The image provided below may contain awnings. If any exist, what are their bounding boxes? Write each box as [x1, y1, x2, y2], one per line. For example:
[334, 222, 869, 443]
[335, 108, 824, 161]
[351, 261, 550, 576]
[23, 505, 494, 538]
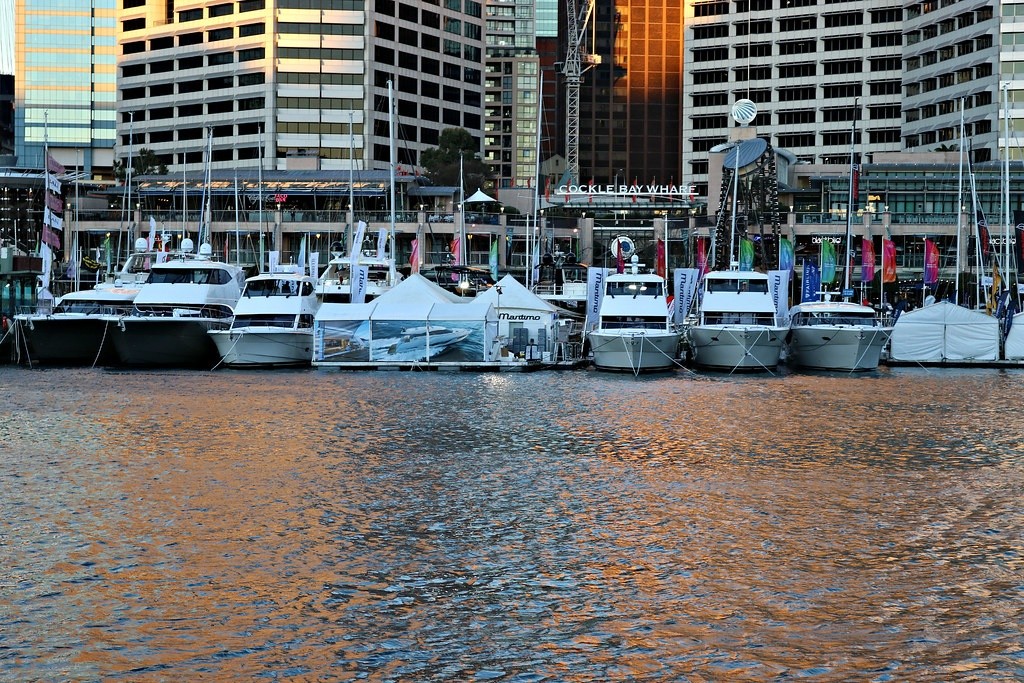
[136, 181, 392, 194]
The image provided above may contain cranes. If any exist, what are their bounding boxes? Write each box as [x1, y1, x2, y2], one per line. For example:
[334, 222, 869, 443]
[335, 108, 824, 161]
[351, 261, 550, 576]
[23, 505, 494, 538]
[560, 0, 604, 191]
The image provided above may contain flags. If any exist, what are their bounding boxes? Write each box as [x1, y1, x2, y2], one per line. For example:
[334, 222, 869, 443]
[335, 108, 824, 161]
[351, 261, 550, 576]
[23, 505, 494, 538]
[42, 153, 63, 291]
[67, 244, 101, 277]
[495, 176, 695, 203]
[224, 238, 228, 256]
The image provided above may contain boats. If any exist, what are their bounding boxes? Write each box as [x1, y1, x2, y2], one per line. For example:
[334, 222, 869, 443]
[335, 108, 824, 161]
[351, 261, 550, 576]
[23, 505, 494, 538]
[585, 270, 678, 372]
[206, 274, 322, 367]
[98, 256, 243, 372]
[324, 334, 361, 358]
[389, 325, 472, 355]
[684, 143, 791, 369]
[315, 256, 405, 300]
[785, 300, 895, 373]
[12, 242, 209, 364]
[532, 253, 588, 312]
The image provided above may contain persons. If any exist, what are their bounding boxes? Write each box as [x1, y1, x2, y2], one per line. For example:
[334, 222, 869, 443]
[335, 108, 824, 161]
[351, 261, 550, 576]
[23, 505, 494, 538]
[281, 281, 313, 297]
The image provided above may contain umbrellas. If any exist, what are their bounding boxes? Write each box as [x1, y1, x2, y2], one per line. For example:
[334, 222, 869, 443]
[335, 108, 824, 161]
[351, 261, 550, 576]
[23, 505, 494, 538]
[460, 187, 500, 203]
